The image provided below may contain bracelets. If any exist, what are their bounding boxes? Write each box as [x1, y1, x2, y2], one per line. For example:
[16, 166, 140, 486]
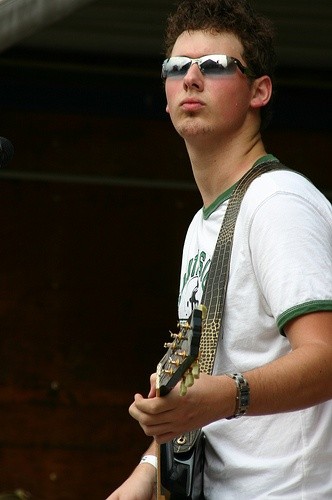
[139, 455, 158, 470]
[224, 372, 251, 421]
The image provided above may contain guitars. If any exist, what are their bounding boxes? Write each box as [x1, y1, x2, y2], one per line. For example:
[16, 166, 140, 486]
[155, 309, 202, 500]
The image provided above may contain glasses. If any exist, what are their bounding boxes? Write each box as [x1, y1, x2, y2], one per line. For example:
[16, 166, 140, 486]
[161, 54, 256, 79]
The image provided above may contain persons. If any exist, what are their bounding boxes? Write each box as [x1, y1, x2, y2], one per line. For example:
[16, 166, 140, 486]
[104, 0, 332, 500]
